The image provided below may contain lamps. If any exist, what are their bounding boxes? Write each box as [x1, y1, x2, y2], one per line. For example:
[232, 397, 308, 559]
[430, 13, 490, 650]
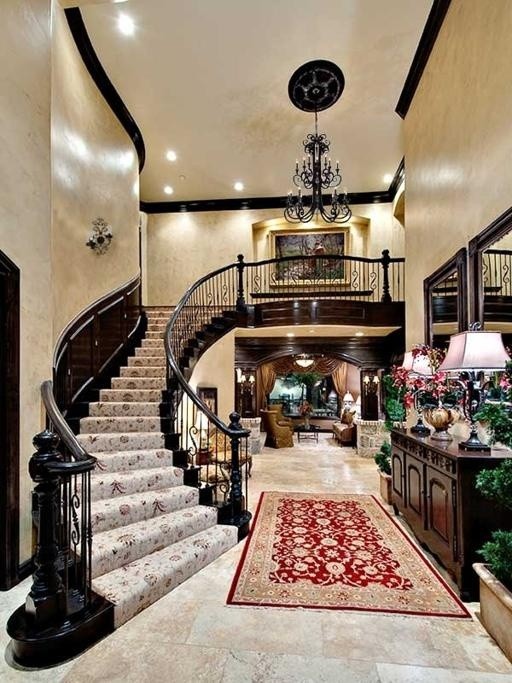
[236, 368, 255, 396]
[194, 410, 210, 451]
[436, 321, 512, 452]
[294, 354, 316, 368]
[343, 389, 355, 407]
[85, 215, 113, 254]
[363, 375, 370, 396]
[401, 350, 436, 436]
[284, 58, 352, 224]
[372, 376, 380, 393]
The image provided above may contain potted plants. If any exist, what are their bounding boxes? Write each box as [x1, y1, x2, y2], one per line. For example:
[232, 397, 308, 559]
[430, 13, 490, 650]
[373, 440, 392, 505]
[471, 457, 512, 665]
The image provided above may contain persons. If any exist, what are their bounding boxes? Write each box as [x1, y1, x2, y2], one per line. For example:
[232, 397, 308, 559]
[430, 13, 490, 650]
[275, 237, 344, 278]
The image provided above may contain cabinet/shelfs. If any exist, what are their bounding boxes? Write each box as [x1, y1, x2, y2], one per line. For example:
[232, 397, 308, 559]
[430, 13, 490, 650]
[389, 429, 512, 603]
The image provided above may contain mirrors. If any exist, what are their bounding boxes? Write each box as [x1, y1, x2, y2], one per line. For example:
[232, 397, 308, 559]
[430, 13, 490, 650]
[469, 204, 512, 358]
[423, 246, 468, 357]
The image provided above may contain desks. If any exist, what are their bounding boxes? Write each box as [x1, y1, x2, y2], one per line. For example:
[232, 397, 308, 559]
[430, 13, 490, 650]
[196, 449, 214, 465]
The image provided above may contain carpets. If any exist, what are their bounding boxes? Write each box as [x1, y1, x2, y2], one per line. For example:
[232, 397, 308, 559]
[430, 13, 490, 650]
[227, 491, 472, 620]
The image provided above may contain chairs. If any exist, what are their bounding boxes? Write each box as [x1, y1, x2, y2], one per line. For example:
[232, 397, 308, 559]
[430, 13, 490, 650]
[208, 426, 252, 479]
[260, 409, 294, 449]
[267, 404, 294, 435]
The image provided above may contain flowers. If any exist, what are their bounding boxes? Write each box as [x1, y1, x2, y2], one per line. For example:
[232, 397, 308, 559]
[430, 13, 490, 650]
[390, 344, 512, 409]
[297, 401, 313, 417]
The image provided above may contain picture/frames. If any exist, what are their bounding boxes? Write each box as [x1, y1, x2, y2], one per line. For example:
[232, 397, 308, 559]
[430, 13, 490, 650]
[269, 225, 352, 288]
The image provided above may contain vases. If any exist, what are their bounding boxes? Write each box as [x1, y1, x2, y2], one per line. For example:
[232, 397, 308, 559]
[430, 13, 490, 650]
[423, 407, 454, 442]
[304, 415, 311, 431]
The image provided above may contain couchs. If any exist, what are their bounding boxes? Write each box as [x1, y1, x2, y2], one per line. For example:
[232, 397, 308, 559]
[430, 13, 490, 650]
[332, 412, 355, 449]
